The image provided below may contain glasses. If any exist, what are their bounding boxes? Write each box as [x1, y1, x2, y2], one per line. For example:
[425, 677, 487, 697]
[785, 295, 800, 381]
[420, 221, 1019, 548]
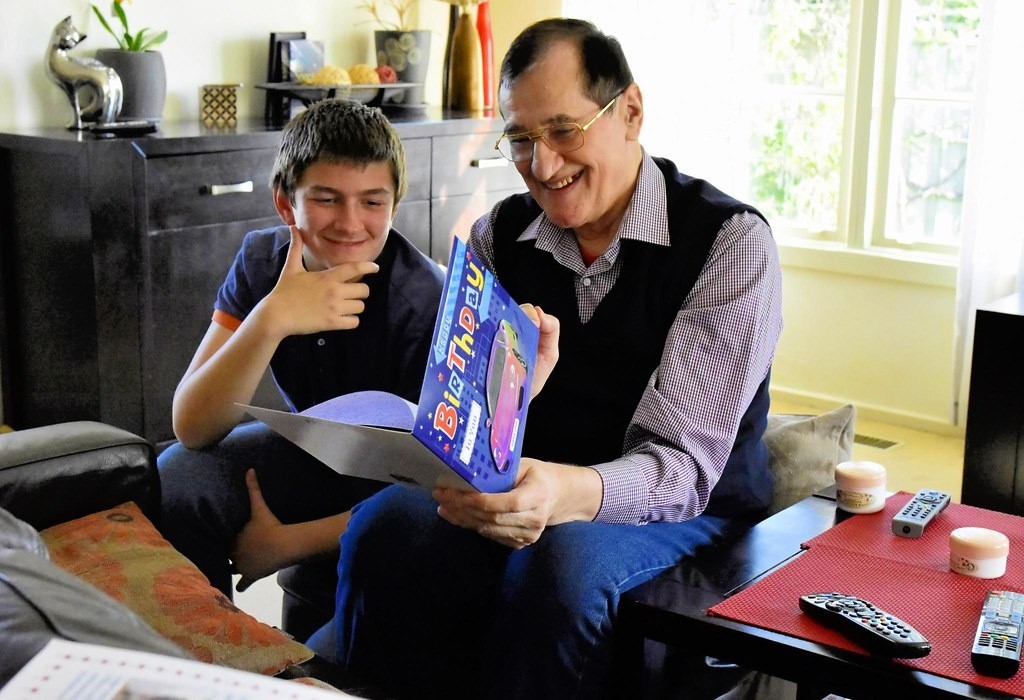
[495, 90, 624, 162]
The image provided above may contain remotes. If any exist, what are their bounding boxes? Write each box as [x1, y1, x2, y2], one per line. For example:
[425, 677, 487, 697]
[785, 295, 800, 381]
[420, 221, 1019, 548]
[971, 591, 1024, 679]
[799, 593, 932, 660]
[891, 489, 952, 537]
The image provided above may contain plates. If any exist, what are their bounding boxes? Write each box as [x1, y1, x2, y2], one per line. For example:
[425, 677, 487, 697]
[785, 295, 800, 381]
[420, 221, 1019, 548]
[255, 81, 421, 106]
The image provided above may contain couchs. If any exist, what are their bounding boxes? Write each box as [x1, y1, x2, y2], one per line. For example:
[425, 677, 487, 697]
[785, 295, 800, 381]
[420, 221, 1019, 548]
[0, 421, 303, 699]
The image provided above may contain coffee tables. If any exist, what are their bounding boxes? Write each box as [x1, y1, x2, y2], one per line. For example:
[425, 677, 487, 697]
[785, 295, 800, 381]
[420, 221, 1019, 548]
[619, 484, 1024, 700]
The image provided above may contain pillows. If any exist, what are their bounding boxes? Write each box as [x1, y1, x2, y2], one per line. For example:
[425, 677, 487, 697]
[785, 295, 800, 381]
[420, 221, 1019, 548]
[763, 403, 857, 516]
[42, 500, 317, 676]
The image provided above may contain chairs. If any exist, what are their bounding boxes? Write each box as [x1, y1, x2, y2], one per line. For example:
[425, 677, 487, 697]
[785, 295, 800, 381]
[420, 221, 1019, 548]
[958, 309, 1024, 518]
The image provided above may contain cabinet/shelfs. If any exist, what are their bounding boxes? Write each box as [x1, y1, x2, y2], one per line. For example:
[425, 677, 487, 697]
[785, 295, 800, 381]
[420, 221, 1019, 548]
[0, 115, 531, 444]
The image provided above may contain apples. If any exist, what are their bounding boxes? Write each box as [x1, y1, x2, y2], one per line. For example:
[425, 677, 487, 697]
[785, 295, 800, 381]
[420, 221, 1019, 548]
[375, 64, 397, 83]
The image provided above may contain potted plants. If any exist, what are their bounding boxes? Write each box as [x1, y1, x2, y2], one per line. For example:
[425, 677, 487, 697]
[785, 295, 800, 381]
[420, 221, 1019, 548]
[90, 0, 168, 123]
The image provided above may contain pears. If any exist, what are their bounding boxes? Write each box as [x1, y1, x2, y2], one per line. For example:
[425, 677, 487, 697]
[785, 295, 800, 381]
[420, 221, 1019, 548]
[311, 64, 351, 86]
[348, 64, 380, 84]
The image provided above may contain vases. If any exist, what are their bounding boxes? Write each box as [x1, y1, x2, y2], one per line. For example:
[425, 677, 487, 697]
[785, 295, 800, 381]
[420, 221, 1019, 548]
[374, 31, 435, 113]
[448, 12, 485, 109]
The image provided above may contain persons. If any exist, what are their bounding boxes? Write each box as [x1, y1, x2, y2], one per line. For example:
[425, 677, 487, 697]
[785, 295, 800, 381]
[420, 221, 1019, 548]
[337, 19, 783, 700]
[158, 99, 445, 644]
